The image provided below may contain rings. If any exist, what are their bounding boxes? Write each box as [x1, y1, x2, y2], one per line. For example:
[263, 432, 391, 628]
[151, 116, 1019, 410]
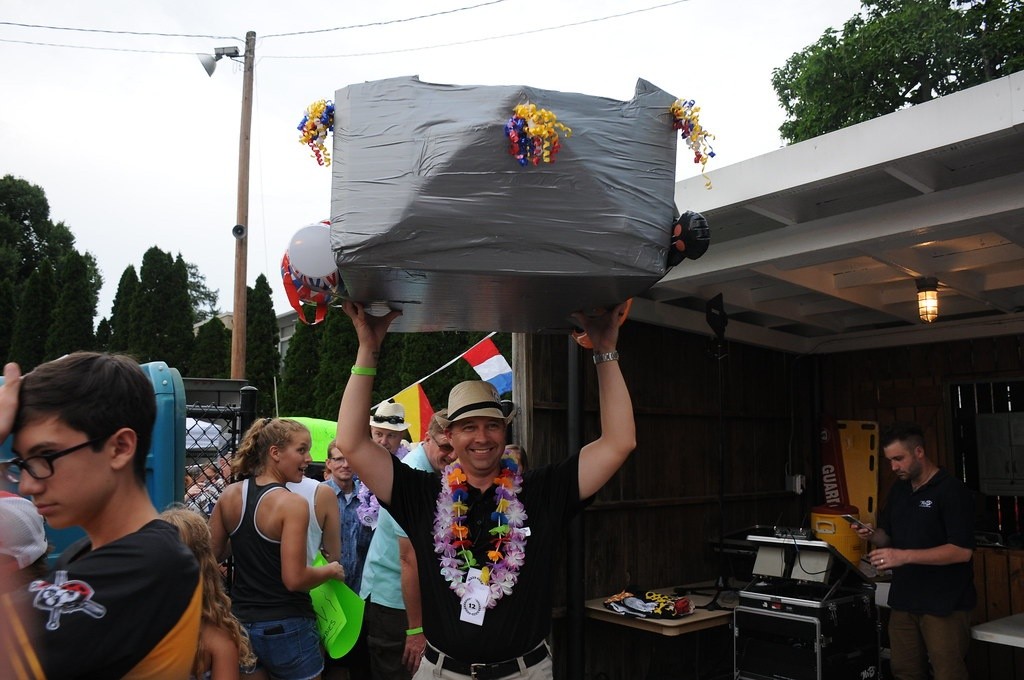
[881, 559, 883, 563]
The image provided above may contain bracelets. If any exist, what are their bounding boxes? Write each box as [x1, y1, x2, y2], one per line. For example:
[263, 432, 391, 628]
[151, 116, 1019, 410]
[593, 351, 619, 363]
[406, 627, 423, 636]
[350, 365, 377, 376]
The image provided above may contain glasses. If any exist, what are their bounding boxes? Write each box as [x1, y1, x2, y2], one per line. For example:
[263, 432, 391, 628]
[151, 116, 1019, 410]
[0, 429, 143, 484]
[428, 432, 454, 453]
[330, 456, 345, 465]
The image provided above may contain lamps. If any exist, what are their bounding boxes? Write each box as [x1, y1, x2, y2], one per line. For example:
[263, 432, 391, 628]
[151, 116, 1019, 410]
[914, 276, 940, 323]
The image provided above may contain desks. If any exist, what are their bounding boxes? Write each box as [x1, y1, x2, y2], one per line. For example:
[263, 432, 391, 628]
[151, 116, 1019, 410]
[970, 613, 1024, 649]
[586, 578, 749, 680]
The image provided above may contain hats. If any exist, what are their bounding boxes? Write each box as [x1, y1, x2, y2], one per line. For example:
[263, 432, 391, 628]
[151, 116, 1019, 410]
[0, 491, 48, 578]
[370, 400, 411, 431]
[434, 380, 516, 430]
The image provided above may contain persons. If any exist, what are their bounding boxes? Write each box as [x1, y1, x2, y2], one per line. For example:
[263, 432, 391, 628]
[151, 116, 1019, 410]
[187, 437, 238, 519]
[335, 301, 636, 680]
[0, 352, 203, 680]
[285, 475, 341, 567]
[208, 416, 345, 680]
[851, 424, 978, 680]
[359, 408, 459, 680]
[320, 439, 361, 584]
[357, 401, 411, 592]
[161, 510, 258, 680]
[506, 444, 528, 474]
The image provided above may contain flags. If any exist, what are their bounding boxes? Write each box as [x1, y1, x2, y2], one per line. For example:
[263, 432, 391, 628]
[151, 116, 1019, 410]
[463, 336, 512, 395]
[394, 383, 435, 443]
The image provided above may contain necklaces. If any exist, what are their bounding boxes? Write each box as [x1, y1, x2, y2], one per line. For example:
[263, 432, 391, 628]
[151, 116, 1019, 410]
[434, 449, 528, 609]
[356, 445, 408, 526]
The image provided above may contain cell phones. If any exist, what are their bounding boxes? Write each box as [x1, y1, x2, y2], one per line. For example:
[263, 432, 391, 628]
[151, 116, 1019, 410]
[263, 624, 285, 636]
[841, 514, 875, 533]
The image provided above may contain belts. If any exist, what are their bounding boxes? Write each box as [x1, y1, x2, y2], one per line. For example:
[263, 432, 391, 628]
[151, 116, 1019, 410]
[423, 640, 550, 680]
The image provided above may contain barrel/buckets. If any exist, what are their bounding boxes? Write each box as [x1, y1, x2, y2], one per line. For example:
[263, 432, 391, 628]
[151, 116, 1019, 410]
[811, 512, 867, 568]
